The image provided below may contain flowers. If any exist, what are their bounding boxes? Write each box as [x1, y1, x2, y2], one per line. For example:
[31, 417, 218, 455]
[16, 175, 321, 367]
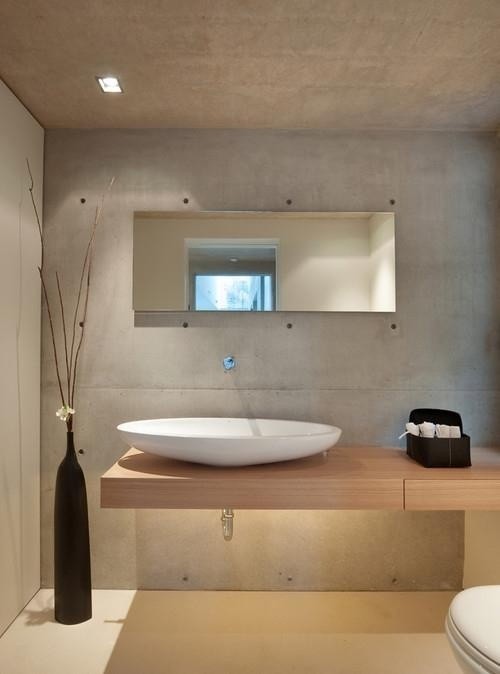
[29, 157, 117, 425]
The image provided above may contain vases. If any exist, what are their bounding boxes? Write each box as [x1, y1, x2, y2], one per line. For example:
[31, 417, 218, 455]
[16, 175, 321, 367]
[54, 432, 91, 625]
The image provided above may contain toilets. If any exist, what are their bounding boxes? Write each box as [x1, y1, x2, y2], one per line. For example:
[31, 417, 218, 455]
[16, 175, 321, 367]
[444, 583, 500, 674]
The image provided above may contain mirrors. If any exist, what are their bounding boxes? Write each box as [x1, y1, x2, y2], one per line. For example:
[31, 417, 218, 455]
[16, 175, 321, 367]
[132, 211, 396, 313]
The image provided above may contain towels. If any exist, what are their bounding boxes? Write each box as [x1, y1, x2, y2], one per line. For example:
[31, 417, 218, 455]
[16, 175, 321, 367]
[405, 421, 461, 439]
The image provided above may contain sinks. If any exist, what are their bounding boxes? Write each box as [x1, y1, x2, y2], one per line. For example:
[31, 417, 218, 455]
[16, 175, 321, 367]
[116, 417, 343, 467]
